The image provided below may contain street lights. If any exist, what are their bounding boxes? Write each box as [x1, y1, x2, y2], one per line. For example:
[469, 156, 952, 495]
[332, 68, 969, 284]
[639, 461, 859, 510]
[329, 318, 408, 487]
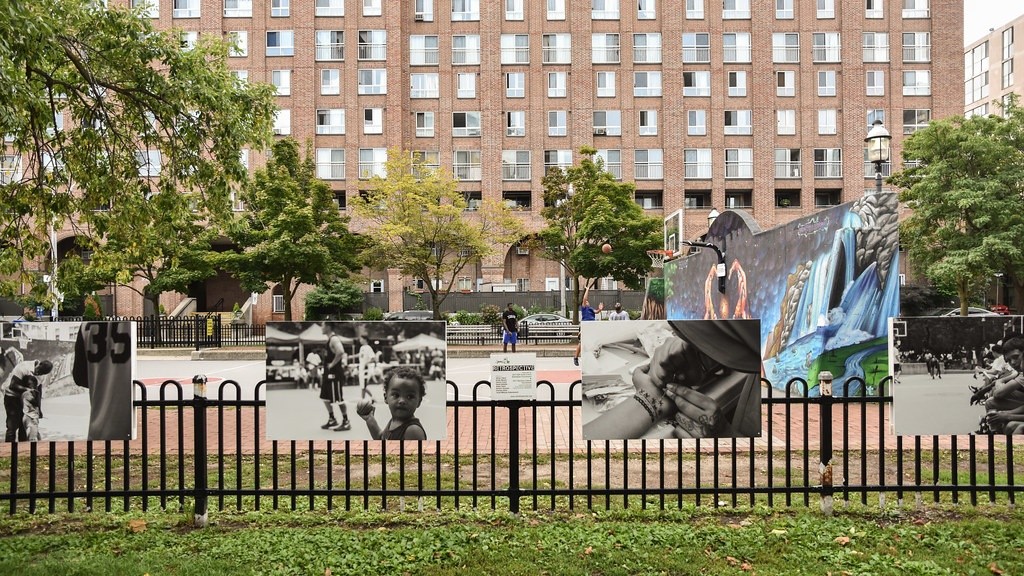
[864, 119, 892, 194]
[993, 266, 1004, 319]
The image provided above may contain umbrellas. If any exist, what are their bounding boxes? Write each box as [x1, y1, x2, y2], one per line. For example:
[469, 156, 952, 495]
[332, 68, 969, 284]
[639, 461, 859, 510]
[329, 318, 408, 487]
[393, 333, 445, 355]
[299, 323, 354, 345]
[266, 325, 300, 348]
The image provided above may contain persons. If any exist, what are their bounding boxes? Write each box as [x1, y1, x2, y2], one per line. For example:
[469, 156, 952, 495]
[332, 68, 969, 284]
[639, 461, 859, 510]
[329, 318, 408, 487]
[649, 320, 761, 439]
[72, 322, 132, 440]
[608, 302, 630, 320]
[320, 322, 351, 432]
[894, 333, 1024, 435]
[356, 367, 427, 440]
[306, 349, 322, 391]
[1, 359, 53, 442]
[400, 346, 444, 382]
[21, 377, 43, 442]
[294, 363, 307, 389]
[583, 363, 691, 439]
[574, 287, 603, 366]
[502, 303, 519, 353]
[347, 334, 377, 404]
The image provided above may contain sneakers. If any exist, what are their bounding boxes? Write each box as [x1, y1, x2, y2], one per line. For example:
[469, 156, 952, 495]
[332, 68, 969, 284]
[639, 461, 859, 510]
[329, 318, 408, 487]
[320, 419, 352, 431]
[574, 357, 579, 366]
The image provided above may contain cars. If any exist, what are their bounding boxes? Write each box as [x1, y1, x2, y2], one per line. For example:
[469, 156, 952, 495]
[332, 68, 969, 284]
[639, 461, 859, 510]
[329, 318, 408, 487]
[386, 311, 435, 323]
[987, 303, 1009, 316]
[500, 314, 574, 337]
[930, 307, 1000, 319]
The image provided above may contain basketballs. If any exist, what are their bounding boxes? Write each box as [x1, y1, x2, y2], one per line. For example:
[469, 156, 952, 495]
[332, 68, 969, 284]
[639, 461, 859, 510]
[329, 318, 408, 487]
[602, 244, 611, 254]
